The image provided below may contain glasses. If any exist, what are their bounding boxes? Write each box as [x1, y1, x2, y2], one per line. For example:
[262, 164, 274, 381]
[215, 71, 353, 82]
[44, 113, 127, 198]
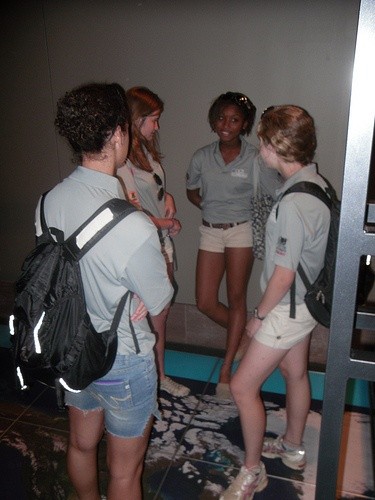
[227, 91, 250, 113]
[153, 173, 165, 202]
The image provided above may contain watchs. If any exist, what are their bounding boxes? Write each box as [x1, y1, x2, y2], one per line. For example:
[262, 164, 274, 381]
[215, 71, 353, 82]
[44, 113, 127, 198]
[253, 307, 265, 321]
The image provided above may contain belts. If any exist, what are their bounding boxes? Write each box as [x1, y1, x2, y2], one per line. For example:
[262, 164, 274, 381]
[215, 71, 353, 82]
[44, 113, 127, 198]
[201, 219, 249, 229]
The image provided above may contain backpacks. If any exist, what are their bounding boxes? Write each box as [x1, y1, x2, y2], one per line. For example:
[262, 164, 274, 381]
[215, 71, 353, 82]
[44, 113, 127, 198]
[274, 171, 374, 328]
[6, 190, 152, 396]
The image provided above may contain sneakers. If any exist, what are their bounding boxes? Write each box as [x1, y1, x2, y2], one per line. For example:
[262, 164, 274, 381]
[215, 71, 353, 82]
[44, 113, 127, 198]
[262, 435, 307, 470]
[220, 460, 269, 500]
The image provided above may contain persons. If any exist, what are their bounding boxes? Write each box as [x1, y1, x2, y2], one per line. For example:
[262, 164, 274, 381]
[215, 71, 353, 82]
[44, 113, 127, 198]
[123, 86, 190, 398]
[220, 105, 334, 500]
[35, 82, 175, 500]
[186, 92, 280, 401]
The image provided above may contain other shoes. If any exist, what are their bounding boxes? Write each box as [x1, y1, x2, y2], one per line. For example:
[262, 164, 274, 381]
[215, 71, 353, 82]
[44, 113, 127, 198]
[215, 382, 232, 404]
[160, 377, 191, 397]
